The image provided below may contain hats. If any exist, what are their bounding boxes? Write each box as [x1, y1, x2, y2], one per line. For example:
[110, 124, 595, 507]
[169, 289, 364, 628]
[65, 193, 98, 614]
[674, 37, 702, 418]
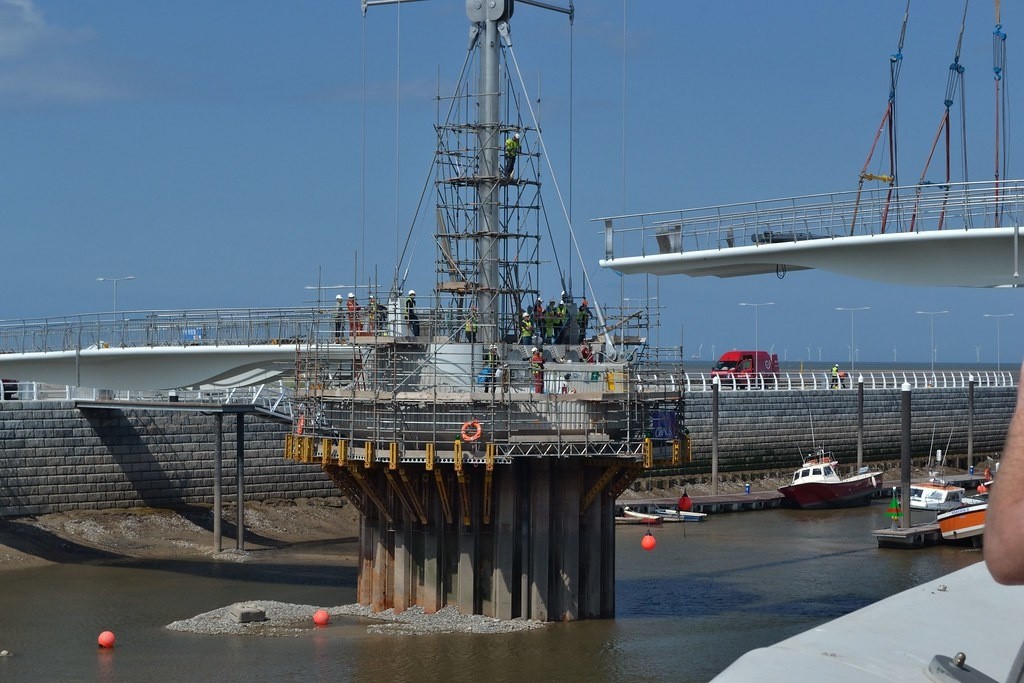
[582, 300, 588, 305]
[369, 295, 374, 298]
[549, 299, 555, 302]
[348, 293, 354, 297]
[489, 345, 497, 350]
[336, 295, 342, 299]
[538, 298, 542, 302]
[523, 312, 530, 318]
[531, 347, 538, 352]
[560, 300, 564, 304]
[473, 305, 476, 309]
[408, 289, 415, 295]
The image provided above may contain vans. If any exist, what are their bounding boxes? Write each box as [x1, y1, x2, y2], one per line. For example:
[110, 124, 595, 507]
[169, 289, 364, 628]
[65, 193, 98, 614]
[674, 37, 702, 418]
[708, 351, 779, 391]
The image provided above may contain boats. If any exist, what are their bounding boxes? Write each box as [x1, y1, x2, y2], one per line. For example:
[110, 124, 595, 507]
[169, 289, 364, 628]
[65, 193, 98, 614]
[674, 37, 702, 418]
[654, 509, 708, 522]
[615, 517, 642, 524]
[777, 446, 883, 509]
[936, 493, 988, 540]
[623, 510, 664, 524]
[910, 478, 965, 511]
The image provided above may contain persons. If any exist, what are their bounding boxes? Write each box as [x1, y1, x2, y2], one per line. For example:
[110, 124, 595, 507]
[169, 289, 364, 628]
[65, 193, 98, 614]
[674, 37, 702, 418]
[465, 305, 479, 343]
[532, 348, 543, 393]
[370, 300, 378, 335]
[577, 301, 594, 343]
[502, 134, 522, 177]
[347, 293, 361, 335]
[483, 345, 500, 393]
[534, 298, 571, 344]
[334, 295, 346, 343]
[521, 313, 534, 344]
[831, 364, 847, 388]
[984, 360, 1024, 586]
[404, 290, 420, 336]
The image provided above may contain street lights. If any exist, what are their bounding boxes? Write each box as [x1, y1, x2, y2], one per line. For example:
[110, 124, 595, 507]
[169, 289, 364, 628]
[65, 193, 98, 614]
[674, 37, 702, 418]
[916, 310, 949, 386]
[982, 314, 1015, 385]
[739, 303, 775, 388]
[835, 307, 871, 387]
[96, 277, 136, 346]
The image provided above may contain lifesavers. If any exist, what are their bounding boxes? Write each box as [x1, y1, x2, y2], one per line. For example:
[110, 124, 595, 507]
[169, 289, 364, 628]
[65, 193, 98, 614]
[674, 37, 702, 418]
[461, 421, 482, 441]
[985, 469, 993, 481]
[820, 457, 832, 463]
[807, 459, 818, 465]
[298, 415, 304, 434]
